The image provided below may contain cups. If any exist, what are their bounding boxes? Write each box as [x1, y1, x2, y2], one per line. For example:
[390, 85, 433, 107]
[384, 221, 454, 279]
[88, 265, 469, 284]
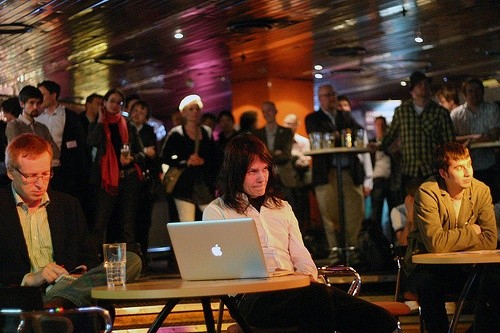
[103, 242, 127, 287]
[322, 132, 335, 148]
[309, 132, 322, 149]
[341, 128, 368, 147]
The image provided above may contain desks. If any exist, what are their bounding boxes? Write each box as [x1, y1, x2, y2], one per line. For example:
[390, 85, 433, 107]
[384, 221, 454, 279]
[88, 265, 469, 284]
[304, 146, 377, 275]
[412, 249, 500, 333]
[90, 273, 310, 333]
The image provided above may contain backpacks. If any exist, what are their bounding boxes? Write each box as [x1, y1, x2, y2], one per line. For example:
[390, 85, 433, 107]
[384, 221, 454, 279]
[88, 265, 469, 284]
[357, 220, 398, 275]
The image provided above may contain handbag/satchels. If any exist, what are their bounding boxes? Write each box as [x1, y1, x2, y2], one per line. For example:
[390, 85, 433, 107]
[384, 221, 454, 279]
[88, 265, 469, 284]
[143, 172, 163, 203]
[162, 166, 182, 194]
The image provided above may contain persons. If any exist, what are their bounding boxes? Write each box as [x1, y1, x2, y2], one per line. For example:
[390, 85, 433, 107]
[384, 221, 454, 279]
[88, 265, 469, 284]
[202, 134, 397, 333]
[402, 141, 500, 333]
[0, 133, 142, 333]
[0, 71, 500, 278]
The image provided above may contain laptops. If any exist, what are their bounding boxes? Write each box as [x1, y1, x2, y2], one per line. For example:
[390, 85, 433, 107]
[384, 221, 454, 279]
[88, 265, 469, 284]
[166, 218, 270, 280]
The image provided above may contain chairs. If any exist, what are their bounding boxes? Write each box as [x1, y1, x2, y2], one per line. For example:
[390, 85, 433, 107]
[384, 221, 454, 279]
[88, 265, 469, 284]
[217, 264, 411, 333]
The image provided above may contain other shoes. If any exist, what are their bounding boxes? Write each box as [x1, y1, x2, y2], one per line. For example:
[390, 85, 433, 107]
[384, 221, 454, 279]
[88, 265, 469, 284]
[348, 249, 360, 260]
[128, 241, 144, 256]
[329, 249, 343, 266]
[147, 255, 169, 268]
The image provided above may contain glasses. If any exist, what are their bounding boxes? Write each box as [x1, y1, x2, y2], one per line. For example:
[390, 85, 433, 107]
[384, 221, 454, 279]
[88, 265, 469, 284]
[12, 166, 54, 182]
[107, 98, 124, 106]
[319, 91, 336, 97]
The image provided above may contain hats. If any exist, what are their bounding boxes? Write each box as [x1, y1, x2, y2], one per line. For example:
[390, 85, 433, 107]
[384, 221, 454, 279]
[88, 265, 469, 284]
[408, 70, 433, 92]
[178, 94, 203, 112]
